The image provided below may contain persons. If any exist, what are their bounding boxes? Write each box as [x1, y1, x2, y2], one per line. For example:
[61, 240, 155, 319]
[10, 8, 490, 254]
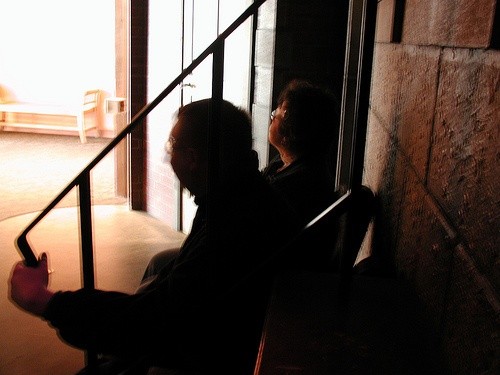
[8, 98, 293, 375]
[140, 80, 339, 284]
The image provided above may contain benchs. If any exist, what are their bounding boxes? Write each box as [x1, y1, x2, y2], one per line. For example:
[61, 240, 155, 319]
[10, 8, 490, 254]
[0, 84, 103, 143]
[252, 184, 378, 375]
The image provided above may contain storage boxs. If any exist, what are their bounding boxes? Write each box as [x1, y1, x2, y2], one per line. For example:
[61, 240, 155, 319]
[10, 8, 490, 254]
[101, 98, 126, 139]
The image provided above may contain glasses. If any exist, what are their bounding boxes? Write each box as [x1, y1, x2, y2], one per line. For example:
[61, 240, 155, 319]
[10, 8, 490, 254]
[164, 141, 183, 160]
[270, 110, 282, 122]
